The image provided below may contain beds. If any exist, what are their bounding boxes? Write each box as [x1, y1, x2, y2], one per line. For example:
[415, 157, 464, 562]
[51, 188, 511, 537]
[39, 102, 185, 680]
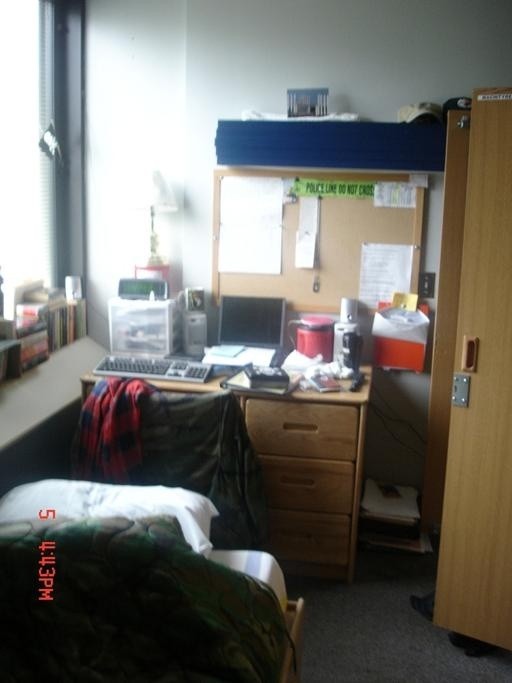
[1, 549, 304, 680]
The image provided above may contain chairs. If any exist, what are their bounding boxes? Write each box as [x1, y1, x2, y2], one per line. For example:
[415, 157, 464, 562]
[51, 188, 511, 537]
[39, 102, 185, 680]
[71, 379, 290, 548]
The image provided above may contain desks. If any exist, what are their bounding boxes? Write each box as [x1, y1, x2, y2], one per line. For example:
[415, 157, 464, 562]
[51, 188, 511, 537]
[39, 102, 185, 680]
[79, 364, 375, 585]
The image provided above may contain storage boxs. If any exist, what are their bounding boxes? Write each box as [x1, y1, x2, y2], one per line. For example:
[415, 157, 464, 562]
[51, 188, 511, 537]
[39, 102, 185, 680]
[107, 298, 183, 356]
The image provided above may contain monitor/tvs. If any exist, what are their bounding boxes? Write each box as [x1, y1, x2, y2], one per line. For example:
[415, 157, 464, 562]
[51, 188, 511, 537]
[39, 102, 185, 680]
[217, 295, 296, 366]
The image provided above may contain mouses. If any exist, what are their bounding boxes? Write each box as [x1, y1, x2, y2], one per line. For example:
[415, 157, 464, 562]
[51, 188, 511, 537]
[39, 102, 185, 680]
[210, 365, 234, 377]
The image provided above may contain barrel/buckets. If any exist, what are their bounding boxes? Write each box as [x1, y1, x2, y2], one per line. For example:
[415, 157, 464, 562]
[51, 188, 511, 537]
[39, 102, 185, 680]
[296, 317, 334, 363]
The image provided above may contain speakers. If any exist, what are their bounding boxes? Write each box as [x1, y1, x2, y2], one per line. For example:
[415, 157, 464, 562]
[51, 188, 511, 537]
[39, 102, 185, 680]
[340, 298, 358, 324]
[180, 310, 209, 361]
[333, 323, 360, 364]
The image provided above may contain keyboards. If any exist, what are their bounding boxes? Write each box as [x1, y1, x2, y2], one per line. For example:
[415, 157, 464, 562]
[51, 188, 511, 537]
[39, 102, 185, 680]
[92, 355, 213, 383]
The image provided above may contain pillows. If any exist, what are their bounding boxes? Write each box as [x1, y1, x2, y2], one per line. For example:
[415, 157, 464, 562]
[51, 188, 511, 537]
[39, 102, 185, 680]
[0, 478, 219, 562]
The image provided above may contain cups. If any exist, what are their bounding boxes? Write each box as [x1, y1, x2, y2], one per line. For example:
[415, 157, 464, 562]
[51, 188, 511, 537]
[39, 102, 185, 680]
[343, 333, 363, 372]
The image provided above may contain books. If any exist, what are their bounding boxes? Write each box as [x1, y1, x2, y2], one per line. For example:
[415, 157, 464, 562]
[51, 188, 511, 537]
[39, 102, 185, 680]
[200, 340, 343, 398]
[0, 269, 88, 383]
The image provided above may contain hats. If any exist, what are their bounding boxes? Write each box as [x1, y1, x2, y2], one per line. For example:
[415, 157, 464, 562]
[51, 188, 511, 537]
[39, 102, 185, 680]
[443, 97, 472, 110]
[399, 103, 443, 125]
